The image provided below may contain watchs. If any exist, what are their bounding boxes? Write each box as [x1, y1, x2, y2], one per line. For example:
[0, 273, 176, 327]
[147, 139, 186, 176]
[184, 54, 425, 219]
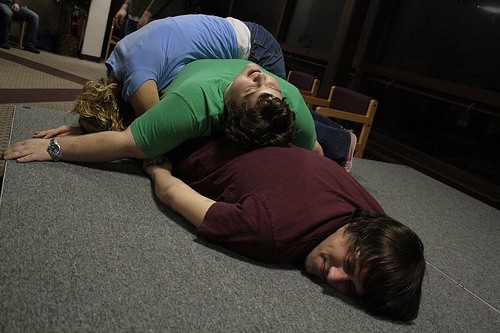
[47, 138, 61, 163]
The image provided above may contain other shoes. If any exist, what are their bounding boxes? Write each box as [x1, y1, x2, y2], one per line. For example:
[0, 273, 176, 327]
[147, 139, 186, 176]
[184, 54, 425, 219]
[0, 45, 10, 50]
[24, 47, 40, 53]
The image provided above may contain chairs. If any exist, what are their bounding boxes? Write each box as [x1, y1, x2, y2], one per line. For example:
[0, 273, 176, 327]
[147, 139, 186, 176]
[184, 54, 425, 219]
[12, 5, 28, 50]
[287, 70, 321, 110]
[302, 85, 378, 158]
[105, 18, 122, 64]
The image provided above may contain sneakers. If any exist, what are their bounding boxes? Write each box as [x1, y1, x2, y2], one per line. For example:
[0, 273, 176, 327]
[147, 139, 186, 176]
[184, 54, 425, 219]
[344, 132, 357, 173]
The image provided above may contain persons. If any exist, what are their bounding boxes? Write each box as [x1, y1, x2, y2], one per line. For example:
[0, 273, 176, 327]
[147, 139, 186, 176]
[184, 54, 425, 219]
[113, 0, 187, 38]
[0, 0, 42, 54]
[5, 14, 425, 311]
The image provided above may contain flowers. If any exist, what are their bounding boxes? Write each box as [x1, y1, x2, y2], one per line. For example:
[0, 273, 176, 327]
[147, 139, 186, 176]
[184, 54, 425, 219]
[55, 0, 88, 34]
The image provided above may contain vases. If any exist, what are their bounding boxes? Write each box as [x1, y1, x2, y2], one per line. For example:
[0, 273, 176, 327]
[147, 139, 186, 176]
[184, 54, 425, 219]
[57, 31, 80, 50]
